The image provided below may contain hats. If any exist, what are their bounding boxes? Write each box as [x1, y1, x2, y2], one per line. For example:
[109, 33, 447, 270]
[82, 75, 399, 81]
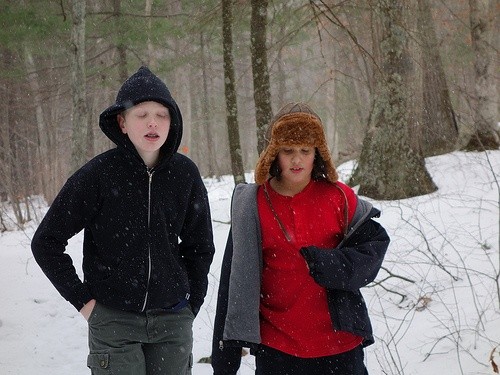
[255, 101, 338, 185]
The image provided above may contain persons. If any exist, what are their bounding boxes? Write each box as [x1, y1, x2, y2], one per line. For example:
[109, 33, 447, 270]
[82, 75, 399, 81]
[211, 102, 392, 374]
[31, 65, 216, 375]
